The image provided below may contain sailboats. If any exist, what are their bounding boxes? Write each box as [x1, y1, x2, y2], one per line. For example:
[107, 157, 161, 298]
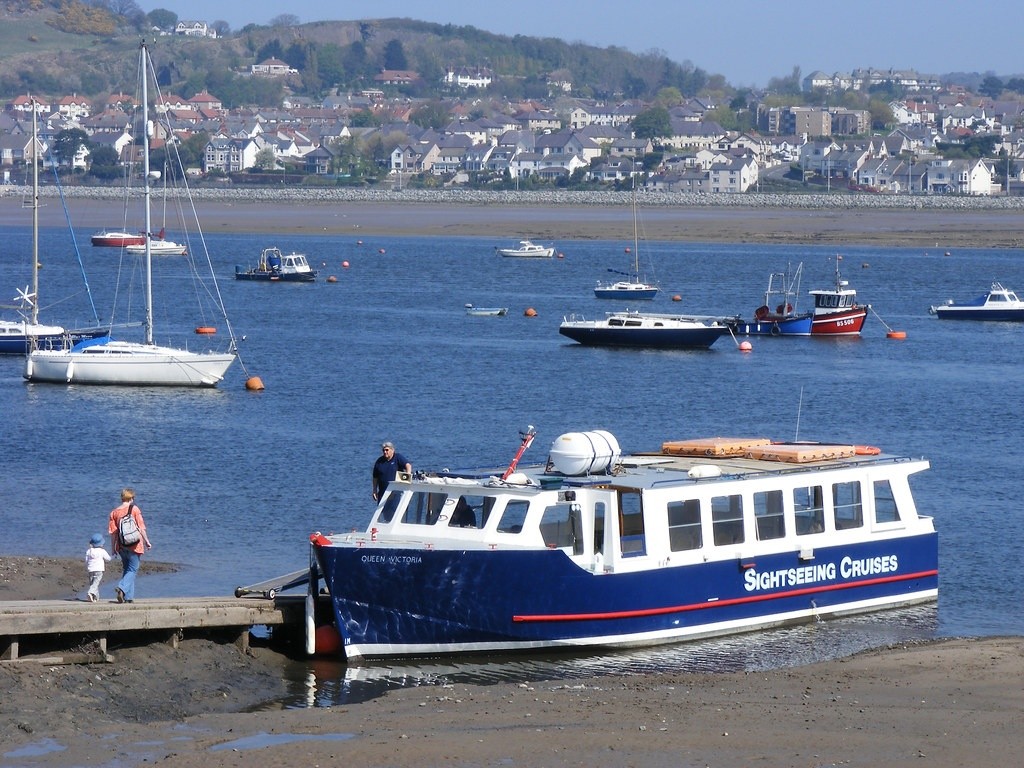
[23, 39, 238, 388]
[593, 189, 660, 299]
[125, 160, 187, 255]
[91, 146, 146, 247]
[0, 95, 110, 355]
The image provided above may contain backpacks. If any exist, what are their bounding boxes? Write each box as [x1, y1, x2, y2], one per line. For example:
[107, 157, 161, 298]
[119, 502, 140, 546]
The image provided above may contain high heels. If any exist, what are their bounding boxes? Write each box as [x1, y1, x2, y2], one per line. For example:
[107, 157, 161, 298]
[125, 600, 132, 603]
[115, 587, 124, 603]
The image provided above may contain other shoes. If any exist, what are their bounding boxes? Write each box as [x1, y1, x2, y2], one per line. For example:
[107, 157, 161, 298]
[87, 593, 94, 602]
[93, 599, 97, 602]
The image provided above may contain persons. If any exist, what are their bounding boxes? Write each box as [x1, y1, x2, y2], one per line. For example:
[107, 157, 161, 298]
[450, 496, 476, 526]
[85, 533, 112, 602]
[108, 488, 151, 603]
[372, 441, 412, 522]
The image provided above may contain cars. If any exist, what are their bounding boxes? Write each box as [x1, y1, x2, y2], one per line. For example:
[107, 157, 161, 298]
[783, 155, 793, 162]
[865, 187, 880, 193]
[848, 185, 863, 191]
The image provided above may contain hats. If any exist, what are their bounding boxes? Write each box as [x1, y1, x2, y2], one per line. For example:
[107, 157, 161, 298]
[90, 534, 105, 548]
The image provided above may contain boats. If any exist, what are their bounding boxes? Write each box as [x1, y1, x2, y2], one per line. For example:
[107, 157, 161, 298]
[499, 241, 556, 258]
[755, 253, 872, 337]
[309, 422, 940, 664]
[559, 310, 745, 350]
[465, 304, 509, 315]
[722, 261, 815, 335]
[929, 281, 1024, 323]
[235, 246, 320, 282]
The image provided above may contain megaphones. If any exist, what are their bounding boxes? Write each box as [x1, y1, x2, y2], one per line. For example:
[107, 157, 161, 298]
[395, 471, 413, 484]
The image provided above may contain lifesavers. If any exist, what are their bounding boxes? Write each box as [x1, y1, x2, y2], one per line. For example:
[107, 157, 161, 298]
[855, 445, 882, 455]
[771, 325, 781, 337]
[852, 303, 857, 310]
[733, 326, 740, 335]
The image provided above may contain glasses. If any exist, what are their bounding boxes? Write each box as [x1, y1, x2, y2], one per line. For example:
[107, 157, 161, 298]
[383, 448, 391, 452]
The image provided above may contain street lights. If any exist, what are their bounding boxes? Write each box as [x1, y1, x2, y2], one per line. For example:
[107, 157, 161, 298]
[803, 155, 810, 182]
